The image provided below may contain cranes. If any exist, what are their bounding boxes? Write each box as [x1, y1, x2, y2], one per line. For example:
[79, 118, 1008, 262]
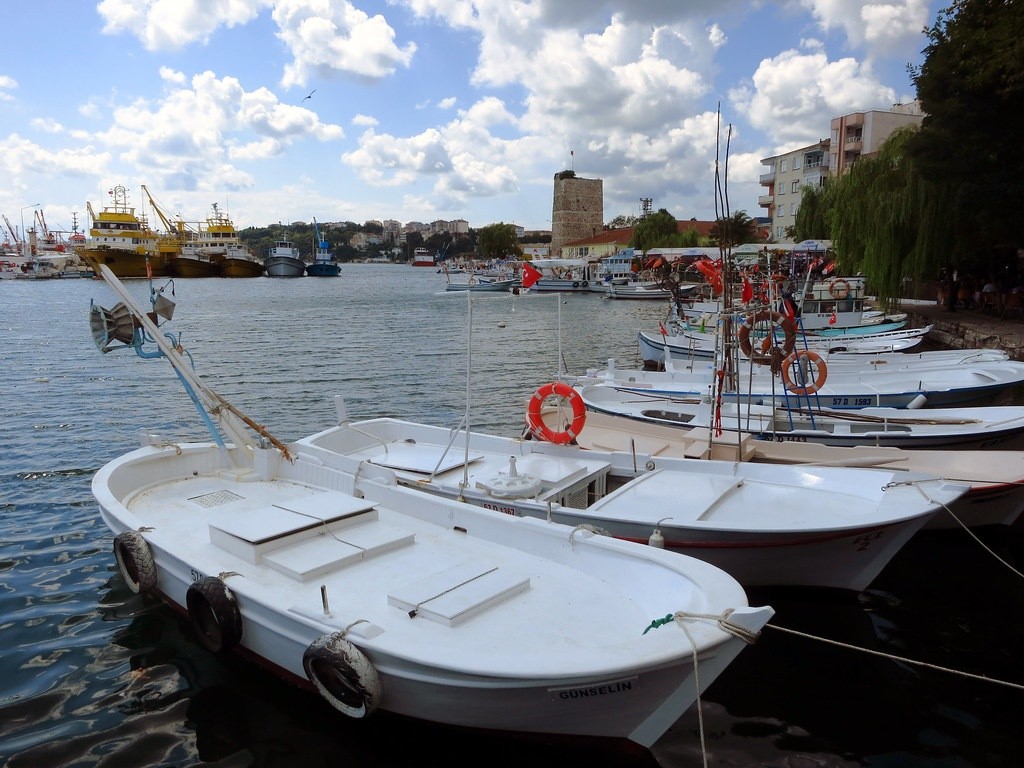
[140, 185, 178, 240]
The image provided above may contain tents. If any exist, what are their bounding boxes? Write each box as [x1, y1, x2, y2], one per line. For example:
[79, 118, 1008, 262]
[644, 239, 839, 264]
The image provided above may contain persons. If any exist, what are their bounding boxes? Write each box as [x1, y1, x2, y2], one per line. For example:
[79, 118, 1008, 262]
[440, 256, 574, 285]
[605, 270, 614, 290]
[633, 250, 840, 283]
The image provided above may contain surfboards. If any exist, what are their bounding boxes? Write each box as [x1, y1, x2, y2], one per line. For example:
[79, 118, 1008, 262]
[777, 406, 982, 425]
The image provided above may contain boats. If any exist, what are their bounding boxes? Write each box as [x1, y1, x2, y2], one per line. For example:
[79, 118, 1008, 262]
[220, 244, 262, 277]
[306, 248, 342, 276]
[531, 281, 590, 290]
[522, 398, 1024, 527]
[75, 184, 168, 276]
[90, 443, 776, 748]
[611, 285, 697, 299]
[437, 261, 509, 277]
[264, 242, 306, 276]
[293, 394, 971, 592]
[170, 241, 217, 278]
[169, 203, 239, 241]
[446, 280, 513, 291]
[578, 276, 1024, 444]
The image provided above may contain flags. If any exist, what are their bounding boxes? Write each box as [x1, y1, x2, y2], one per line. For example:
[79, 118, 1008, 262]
[522, 262, 544, 287]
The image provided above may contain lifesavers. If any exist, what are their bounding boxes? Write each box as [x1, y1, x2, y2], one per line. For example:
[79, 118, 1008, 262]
[829, 279, 850, 300]
[573, 281, 579, 288]
[582, 281, 588, 286]
[526, 383, 588, 444]
[758, 283, 779, 304]
[739, 311, 797, 367]
[781, 351, 827, 395]
[112, 530, 160, 592]
[183, 576, 246, 653]
[302, 634, 382, 720]
